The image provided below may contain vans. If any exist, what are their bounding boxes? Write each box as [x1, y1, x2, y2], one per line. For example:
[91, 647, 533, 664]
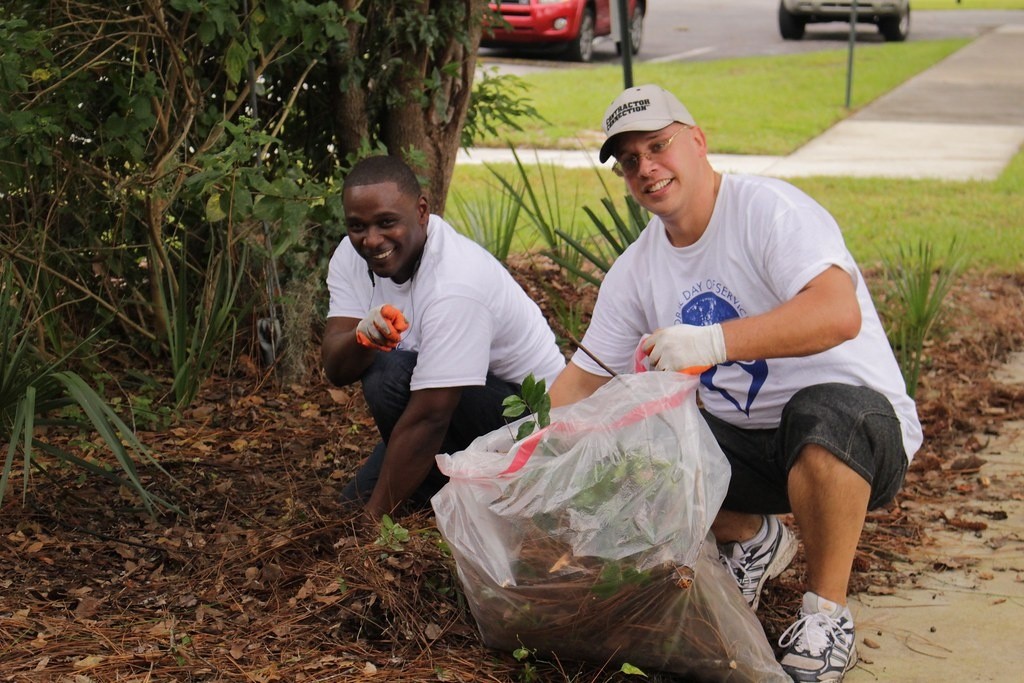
[479, 0, 646, 62]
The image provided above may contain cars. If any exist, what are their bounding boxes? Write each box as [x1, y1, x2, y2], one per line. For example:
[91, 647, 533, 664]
[778, 0, 911, 42]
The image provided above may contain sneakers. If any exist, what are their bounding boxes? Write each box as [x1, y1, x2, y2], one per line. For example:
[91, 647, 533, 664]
[719, 511, 798, 613]
[779, 591, 858, 683]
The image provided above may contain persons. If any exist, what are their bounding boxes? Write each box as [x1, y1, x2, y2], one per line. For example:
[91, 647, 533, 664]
[322, 156, 565, 535]
[548, 82, 922, 683]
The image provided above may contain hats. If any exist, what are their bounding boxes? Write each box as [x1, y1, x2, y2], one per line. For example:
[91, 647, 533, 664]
[600, 84, 699, 163]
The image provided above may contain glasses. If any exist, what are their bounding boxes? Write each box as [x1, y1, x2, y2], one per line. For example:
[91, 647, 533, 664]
[612, 125, 690, 177]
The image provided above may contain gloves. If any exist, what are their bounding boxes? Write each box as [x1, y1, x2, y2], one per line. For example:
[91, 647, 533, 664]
[356, 302, 408, 351]
[641, 323, 726, 376]
